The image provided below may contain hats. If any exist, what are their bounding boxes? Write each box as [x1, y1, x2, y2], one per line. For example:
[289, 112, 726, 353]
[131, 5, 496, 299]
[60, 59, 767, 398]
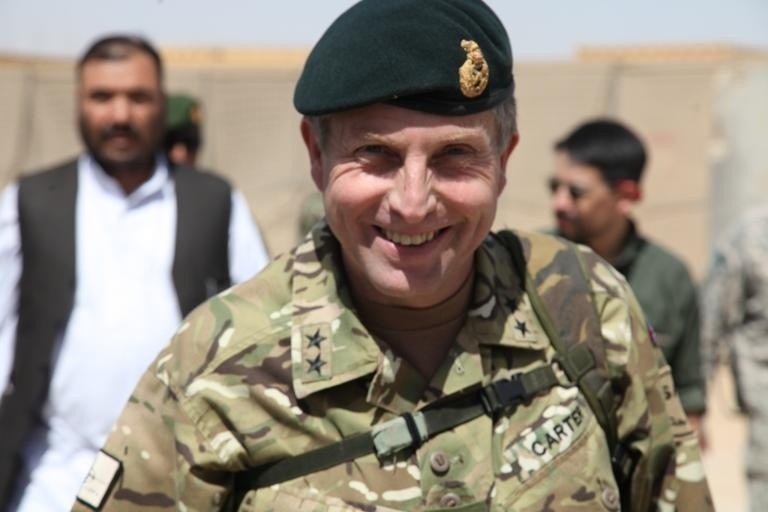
[166, 93, 202, 141]
[293, 0, 515, 118]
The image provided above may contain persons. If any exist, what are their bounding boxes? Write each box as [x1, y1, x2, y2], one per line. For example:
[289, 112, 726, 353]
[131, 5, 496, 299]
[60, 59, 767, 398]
[701, 198, 768, 512]
[72, 0, 698, 512]
[2, 36, 271, 512]
[162, 99, 205, 168]
[539, 118, 707, 459]
